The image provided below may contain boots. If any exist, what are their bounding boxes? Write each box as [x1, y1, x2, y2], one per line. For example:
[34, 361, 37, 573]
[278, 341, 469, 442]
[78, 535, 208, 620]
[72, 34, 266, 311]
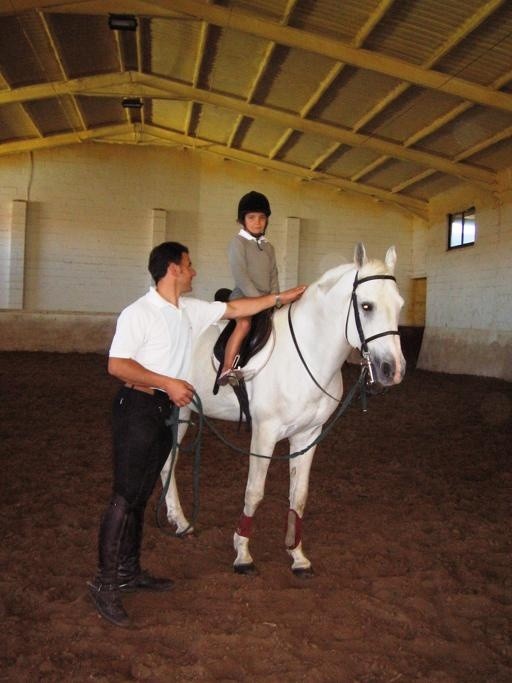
[117, 501, 178, 593]
[87, 492, 132, 628]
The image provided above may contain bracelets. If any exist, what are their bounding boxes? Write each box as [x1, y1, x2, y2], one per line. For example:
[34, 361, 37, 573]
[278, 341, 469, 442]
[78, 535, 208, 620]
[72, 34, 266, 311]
[275, 293, 282, 309]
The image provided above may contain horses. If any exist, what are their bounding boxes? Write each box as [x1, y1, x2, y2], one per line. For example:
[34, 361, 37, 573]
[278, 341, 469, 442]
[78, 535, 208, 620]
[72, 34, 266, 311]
[160, 241, 408, 580]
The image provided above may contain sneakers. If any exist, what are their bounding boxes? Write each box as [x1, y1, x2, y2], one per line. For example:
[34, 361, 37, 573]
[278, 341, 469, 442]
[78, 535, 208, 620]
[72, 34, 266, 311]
[216, 367, 242, 387]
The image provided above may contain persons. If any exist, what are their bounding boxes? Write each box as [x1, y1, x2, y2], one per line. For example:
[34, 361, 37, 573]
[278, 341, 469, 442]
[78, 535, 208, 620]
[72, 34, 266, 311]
[216, 187, 280, 387]
[86, 239, 308, 628]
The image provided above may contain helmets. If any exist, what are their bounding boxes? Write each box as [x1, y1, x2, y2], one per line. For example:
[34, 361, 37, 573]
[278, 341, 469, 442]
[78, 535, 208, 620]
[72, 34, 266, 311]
[237, 190, 271, 221]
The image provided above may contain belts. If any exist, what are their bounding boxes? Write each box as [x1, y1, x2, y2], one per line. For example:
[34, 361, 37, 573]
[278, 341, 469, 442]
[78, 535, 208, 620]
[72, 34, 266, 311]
[124, 383, 154, 397]
